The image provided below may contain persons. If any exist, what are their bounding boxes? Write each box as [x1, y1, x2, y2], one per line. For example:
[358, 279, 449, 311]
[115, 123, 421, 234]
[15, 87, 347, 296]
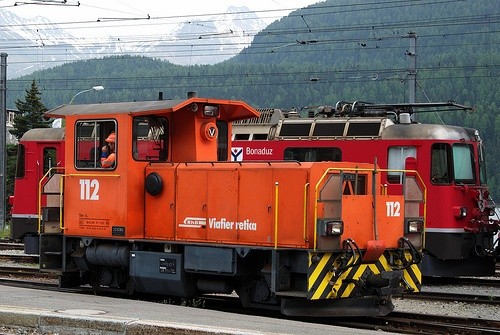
[101, 133, 115, 169]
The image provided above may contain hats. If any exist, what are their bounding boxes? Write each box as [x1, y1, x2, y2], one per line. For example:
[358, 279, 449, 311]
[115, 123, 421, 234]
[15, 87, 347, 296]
[105, 132, 117, 142]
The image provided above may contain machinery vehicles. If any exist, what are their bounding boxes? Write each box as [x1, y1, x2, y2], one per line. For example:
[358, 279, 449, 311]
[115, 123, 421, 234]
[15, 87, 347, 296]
[38, 97, 426, 317]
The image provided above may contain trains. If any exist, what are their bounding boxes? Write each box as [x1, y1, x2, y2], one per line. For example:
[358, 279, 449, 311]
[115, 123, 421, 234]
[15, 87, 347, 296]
[9, 101, 498, 279]
